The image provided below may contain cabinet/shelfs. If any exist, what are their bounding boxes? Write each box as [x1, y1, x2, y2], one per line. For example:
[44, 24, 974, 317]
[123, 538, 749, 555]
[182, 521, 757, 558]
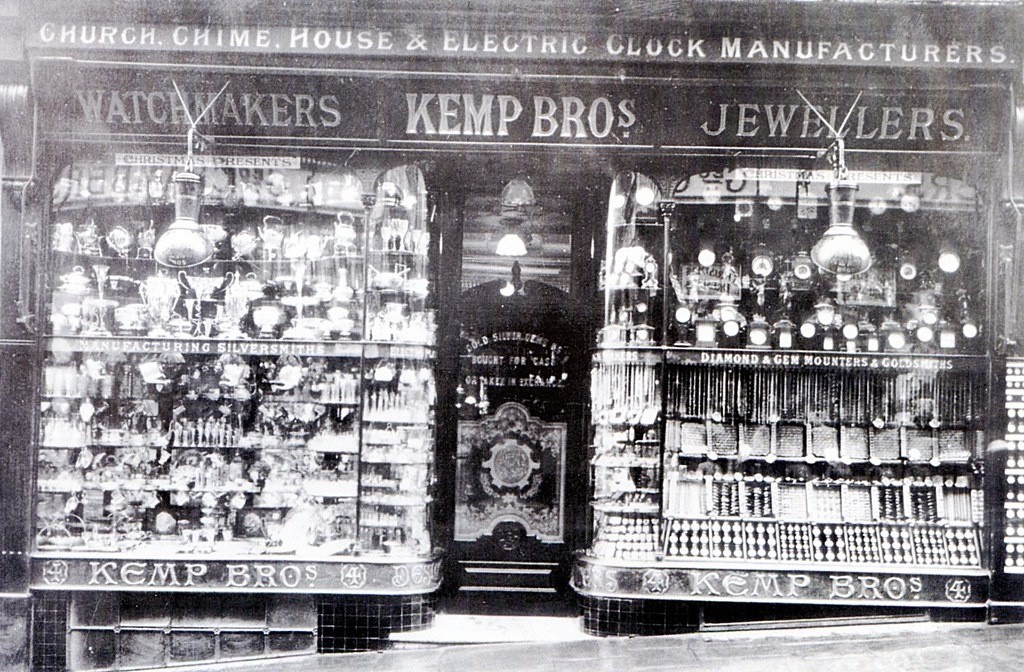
[570, 162, 1000, 609]
[25, 147, 450, 596]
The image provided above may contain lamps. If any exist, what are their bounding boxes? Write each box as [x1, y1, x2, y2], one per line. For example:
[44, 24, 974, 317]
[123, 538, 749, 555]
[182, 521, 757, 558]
[494, 169, 538, 257]
[156, 128, 224, 273]
[810, 134, 874, 277]
